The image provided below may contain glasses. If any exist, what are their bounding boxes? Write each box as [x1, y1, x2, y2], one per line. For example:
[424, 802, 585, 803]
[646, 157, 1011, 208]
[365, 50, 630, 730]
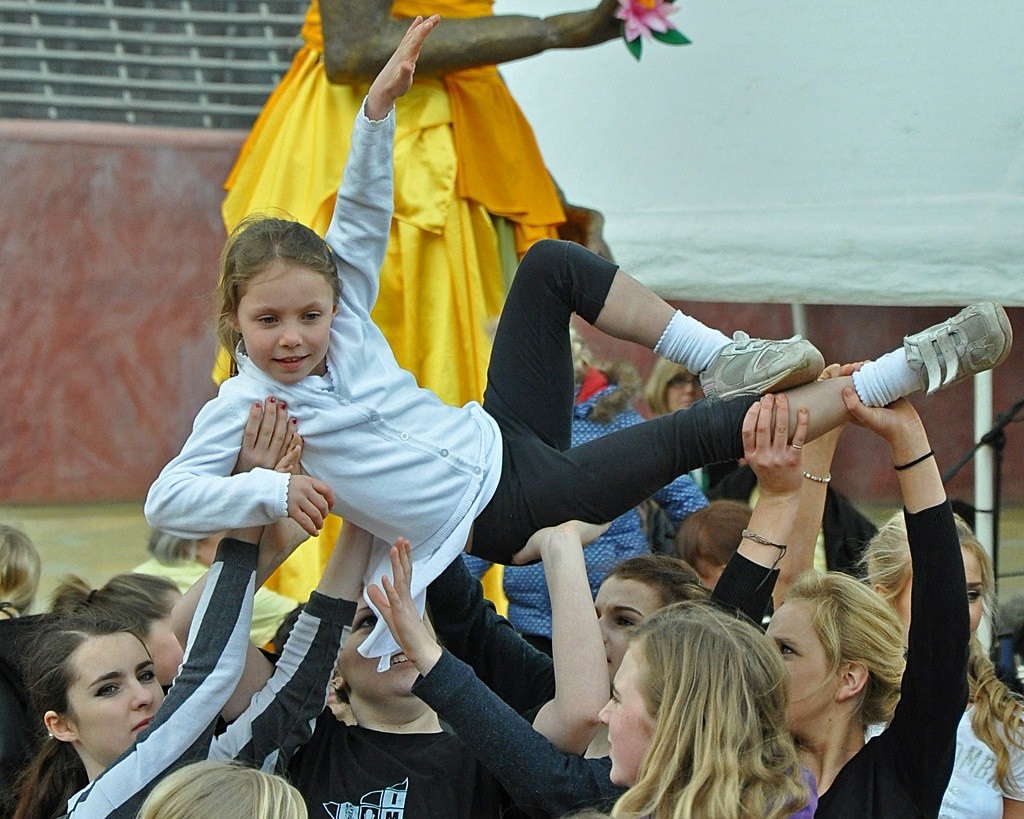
[668, 372, 701, 389]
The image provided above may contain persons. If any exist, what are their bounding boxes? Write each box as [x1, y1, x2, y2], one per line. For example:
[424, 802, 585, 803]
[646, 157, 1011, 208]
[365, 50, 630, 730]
[715, 454, 877, 584]
[0, 397, 754, 819]
[142, 15, 1005, 568]
[597, 601, 818, 819]
[635, 351, 707, 558]
[707, 362, 973, 819]
[771, 361, 1024, 819]
[459, 322, 705, 660]
[211, 1, 690, 617]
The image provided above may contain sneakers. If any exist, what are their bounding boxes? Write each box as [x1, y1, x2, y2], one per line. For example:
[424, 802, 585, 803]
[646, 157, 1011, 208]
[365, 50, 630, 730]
[903, 300, 1013, 396]
[699, 330, 825, 402]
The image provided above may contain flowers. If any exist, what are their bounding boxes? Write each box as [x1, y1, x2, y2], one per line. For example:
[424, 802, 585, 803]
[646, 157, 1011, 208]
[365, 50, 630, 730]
[612, 0, 693, 63]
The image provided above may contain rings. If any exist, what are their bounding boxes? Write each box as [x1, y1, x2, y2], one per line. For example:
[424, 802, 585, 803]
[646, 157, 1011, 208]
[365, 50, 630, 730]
[792, 444, 803, 450]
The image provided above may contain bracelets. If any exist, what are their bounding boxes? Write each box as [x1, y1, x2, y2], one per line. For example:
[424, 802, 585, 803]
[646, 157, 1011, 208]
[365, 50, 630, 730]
[803, 471, 831, 483]
[741, 530, 787, 593]
[893, 449, 935, 470]
